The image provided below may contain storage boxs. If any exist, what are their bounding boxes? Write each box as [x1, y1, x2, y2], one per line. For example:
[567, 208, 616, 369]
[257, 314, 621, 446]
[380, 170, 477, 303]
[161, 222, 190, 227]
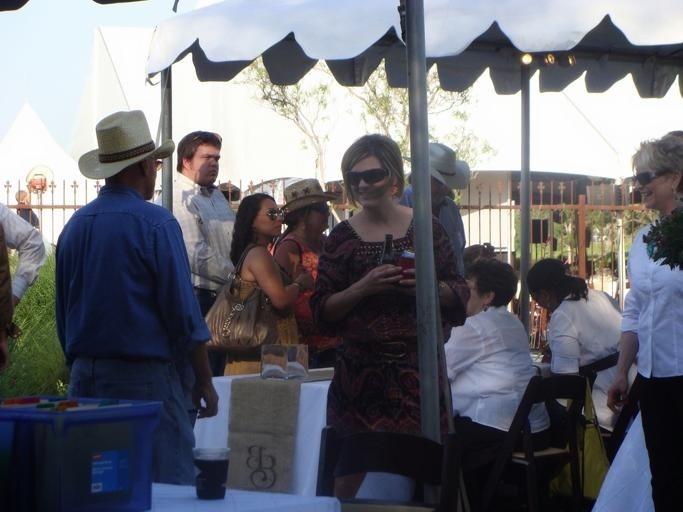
[0, 395, 162, 511]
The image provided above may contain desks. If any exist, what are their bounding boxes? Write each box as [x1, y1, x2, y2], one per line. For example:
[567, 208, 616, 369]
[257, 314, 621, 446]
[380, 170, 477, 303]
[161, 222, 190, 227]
[193, 369, 335, 495]
[150, 481, 340, 512]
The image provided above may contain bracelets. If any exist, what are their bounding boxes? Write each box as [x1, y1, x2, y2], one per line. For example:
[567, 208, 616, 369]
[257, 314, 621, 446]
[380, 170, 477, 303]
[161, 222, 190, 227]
[290, 281, 302, 293]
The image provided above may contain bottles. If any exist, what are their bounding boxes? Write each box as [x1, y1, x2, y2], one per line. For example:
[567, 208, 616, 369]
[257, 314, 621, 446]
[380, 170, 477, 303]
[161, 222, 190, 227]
[379, 233, 397, 268]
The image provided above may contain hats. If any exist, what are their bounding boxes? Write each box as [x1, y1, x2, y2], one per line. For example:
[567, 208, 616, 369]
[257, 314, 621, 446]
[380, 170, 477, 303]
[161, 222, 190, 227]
[402, 142, 469, 189]
[278, 178, 338, 215]
[78, 110, 174, 179]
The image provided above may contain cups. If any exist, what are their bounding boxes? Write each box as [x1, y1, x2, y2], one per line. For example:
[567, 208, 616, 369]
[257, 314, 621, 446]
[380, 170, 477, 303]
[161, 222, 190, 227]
[189, 446, 231, 500]
[396, 249, 416, 277]
[285, 343, 310, 378]
[260, 343, 288, 381]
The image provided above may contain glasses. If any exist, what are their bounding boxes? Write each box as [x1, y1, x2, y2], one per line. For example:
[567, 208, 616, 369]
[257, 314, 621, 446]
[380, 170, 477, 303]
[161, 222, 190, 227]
[309, 205, 328, 213]
[345, 169, 387, 185]
[630, 170, 666, 186]
[147, 156, 163, 171]
[256, 208, 285, 221]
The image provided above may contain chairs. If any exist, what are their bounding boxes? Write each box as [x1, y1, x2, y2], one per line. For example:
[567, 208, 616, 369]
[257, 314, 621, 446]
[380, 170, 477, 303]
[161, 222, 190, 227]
[579, 352, 639, 463]
[454, 375, 588, 510]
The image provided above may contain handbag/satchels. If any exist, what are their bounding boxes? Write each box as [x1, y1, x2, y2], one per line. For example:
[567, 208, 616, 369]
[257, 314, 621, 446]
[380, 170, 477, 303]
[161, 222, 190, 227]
[201, 243, 278, 357]
[547, 377, 610, 500]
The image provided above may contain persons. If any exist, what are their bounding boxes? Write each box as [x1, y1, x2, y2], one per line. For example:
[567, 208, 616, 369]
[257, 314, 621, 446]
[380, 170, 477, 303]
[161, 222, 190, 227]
[52, 108, 219, 488]
[219, 192, 317, 375]
[440, 255, 555, 511]
[0, 202, 45, 378]
[524, 258, 637, 436]
[461, 239, 497, 265]
[14, 188, 39, 232]
[152, 129, 238, 375]
[605, 138, 681, 511]
[308, 132, 473, 501]
[272, 177, 341, 369]
[396, 140, 471, 279]
